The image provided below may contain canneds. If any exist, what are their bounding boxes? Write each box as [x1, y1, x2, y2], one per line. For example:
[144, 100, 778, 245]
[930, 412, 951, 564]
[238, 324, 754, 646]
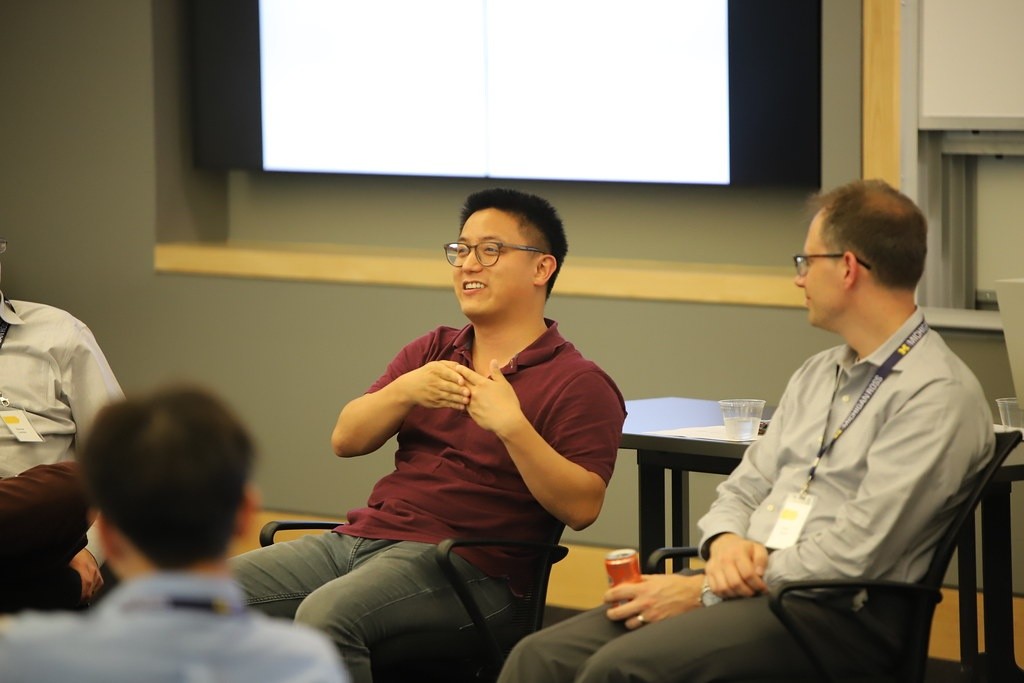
[605, 549, 641, 617]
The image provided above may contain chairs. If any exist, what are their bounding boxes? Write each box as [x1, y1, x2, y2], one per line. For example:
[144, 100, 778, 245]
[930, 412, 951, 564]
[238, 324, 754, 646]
[259, 517, 571, 675]
[640, 428, 1023, 683]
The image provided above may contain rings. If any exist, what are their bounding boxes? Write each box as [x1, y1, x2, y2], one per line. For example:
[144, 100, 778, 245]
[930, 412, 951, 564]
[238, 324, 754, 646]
[636, 614, 647, 625]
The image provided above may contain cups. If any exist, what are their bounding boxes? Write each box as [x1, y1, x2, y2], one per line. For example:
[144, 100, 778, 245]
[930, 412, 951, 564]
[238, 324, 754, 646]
[718, 397, 767, 440]
[995, 396, 1024, 442]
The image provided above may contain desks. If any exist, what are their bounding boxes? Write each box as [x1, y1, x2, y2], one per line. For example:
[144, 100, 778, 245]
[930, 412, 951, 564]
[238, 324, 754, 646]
[613, 415, 1023, 670]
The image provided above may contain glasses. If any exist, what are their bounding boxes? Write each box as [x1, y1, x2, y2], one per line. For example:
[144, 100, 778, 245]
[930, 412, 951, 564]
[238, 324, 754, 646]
[443, 241, 551, 267]
[791, 253, 871, 278]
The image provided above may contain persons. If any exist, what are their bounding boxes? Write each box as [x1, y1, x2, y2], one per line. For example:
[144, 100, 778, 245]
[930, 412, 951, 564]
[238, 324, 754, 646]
[222, 178, 627, 683]
[0, 236, 129, 604]
[0, 382, 354, 682]
[494, 174, 996, 682]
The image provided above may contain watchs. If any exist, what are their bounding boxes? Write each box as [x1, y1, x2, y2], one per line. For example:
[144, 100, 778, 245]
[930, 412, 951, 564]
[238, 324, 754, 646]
[699, 578, 724, 607]
